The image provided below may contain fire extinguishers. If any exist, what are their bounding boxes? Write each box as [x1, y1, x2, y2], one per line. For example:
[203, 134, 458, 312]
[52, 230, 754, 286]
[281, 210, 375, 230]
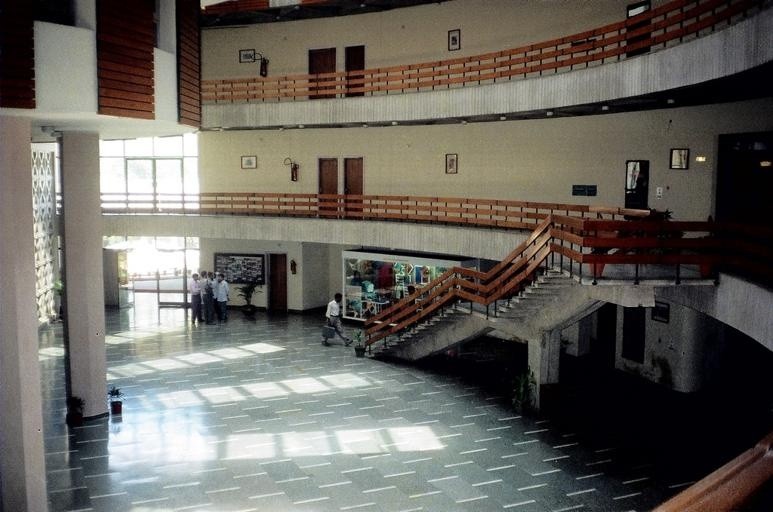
[259, 58, 268, 76]
[291, 164, 299, 181]
[290, 258, 296, 274]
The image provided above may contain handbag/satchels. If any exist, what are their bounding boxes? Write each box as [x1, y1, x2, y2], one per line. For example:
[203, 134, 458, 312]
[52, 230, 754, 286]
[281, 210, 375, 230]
[322, 325, 335, 337]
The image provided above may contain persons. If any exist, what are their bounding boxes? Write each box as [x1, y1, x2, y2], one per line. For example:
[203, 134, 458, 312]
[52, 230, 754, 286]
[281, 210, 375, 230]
[217, 273, 230, 323]
[350, 270, 373, 318]
[207, 272, 221, 321]
[190, 273, 204, 324]
[199, 271, 216, 325]
[320, 292, 353, 347]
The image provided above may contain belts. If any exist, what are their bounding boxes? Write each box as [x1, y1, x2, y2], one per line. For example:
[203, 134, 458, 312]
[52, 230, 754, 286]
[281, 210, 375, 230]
[330, 315, 339, 317]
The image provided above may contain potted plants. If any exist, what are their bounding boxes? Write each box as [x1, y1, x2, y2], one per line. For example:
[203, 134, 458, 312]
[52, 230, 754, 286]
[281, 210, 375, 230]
[233, 274, 262, 317]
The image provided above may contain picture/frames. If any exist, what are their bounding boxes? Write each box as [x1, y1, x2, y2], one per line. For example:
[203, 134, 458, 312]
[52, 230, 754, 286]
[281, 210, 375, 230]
[649, 300, 670, 324]
[240, 155, 257, 169]
[213, 252, 265, 286]
[669, 148, 690, 170]
[447, 29, 461, 52]
[445, 153, 458, 175]
[238, 49, 255, 63]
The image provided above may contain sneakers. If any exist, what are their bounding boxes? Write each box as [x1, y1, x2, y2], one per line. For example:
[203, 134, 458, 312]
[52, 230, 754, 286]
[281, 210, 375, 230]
[344, 339, 353, 345]
[192, 317, 228, 325]
